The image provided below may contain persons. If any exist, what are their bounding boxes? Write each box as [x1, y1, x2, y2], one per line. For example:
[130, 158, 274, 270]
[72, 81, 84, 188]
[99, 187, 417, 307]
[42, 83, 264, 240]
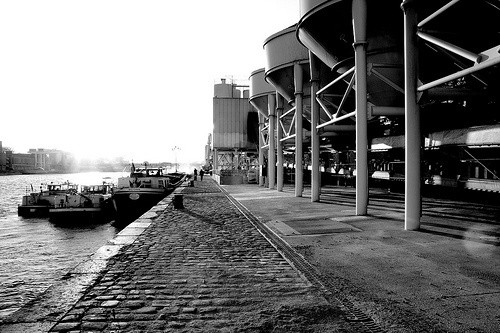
[200, 169, 204, 181]
[194, 168, 197, 181]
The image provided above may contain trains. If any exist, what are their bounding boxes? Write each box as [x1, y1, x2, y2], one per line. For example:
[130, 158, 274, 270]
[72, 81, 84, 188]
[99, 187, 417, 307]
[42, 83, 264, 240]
[275, 124, 500, 197]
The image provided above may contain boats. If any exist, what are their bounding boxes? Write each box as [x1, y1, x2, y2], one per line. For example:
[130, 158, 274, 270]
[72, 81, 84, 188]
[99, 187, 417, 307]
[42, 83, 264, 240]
[50, 181, 113, 227]
[18, 180, 78, 217]
[115, 161, 173, 216]
